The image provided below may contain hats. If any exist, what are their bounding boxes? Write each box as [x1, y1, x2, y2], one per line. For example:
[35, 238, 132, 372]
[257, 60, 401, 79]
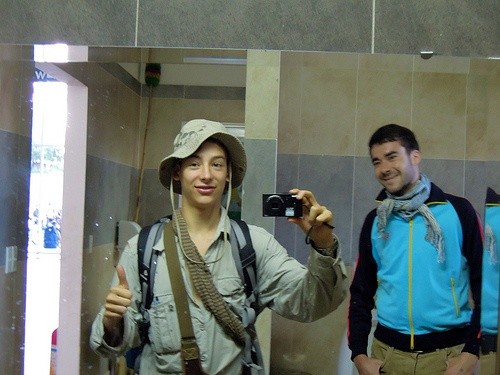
[158, 119, 247, 195]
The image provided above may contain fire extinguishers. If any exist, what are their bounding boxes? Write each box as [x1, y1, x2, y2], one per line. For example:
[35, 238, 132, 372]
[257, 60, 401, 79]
[50, 328, 57, 375]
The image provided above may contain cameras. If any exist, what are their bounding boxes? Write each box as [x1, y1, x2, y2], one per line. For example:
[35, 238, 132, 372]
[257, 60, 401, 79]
[263, 193, 304, 218]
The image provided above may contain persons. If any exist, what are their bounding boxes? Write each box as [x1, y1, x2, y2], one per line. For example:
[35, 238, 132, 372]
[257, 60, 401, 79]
[347, 124, 484, 375]
[90, 119, 348, 375]
[480, 186, 500, 375]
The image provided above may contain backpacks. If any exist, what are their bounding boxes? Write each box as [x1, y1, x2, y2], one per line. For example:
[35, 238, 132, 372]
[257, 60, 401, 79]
[43, 226, 60, 248]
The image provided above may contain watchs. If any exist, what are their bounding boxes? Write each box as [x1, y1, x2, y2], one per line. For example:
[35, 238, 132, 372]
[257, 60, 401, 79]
[316, 240, 338, 255]
